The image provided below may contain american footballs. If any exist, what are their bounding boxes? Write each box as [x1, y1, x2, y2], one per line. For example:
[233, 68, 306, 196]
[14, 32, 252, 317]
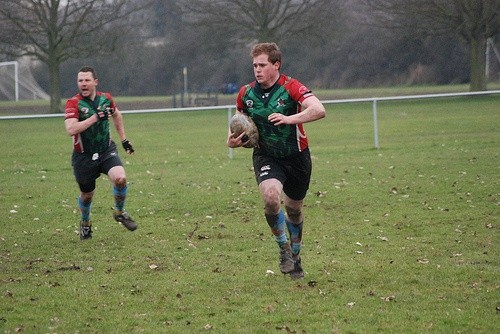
[229, 113, 260, 149]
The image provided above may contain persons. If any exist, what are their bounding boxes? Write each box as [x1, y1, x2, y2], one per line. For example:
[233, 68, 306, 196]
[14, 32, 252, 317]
[227, 42, 326, 281]
[64, 67, 137, 240]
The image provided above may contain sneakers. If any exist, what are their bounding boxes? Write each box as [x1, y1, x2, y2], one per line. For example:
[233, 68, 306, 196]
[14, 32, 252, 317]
[80, 219, 92, 240]
[115, 212, 138, 232]
[280, 249, 294, 273]
[291, 258, 304, 282]
